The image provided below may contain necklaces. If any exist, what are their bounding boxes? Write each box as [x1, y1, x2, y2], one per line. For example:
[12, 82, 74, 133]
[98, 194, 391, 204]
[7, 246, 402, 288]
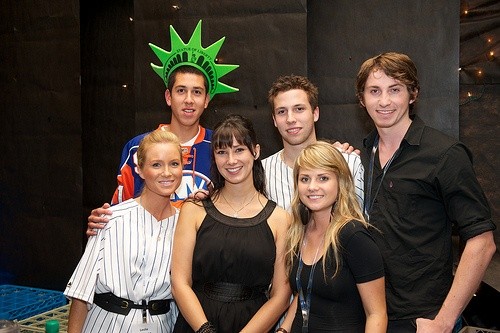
[218, 191, 260, 218]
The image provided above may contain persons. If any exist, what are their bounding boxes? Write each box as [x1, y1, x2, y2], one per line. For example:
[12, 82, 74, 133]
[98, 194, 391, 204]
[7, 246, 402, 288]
[276, 143, 388, 333]
[62, 130, 180, 333]
[188, 73, 364, 214]
[171, 113, 292, 333]
[86, 58, 223, 237]
[335, 51, 496, 332]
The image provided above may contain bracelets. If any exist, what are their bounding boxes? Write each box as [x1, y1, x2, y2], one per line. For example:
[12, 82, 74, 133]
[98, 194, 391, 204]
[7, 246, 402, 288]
[275, 327, 288, 333]
[195, 321, 216, 333]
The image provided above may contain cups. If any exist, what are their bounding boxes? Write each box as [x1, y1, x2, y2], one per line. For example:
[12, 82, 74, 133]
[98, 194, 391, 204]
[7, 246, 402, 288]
[45, 320, 59, 333]
[0, 320, 21, 333]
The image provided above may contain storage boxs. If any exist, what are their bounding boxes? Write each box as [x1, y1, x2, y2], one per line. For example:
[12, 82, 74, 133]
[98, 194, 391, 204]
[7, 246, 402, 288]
[0, 285, 67, 322]
